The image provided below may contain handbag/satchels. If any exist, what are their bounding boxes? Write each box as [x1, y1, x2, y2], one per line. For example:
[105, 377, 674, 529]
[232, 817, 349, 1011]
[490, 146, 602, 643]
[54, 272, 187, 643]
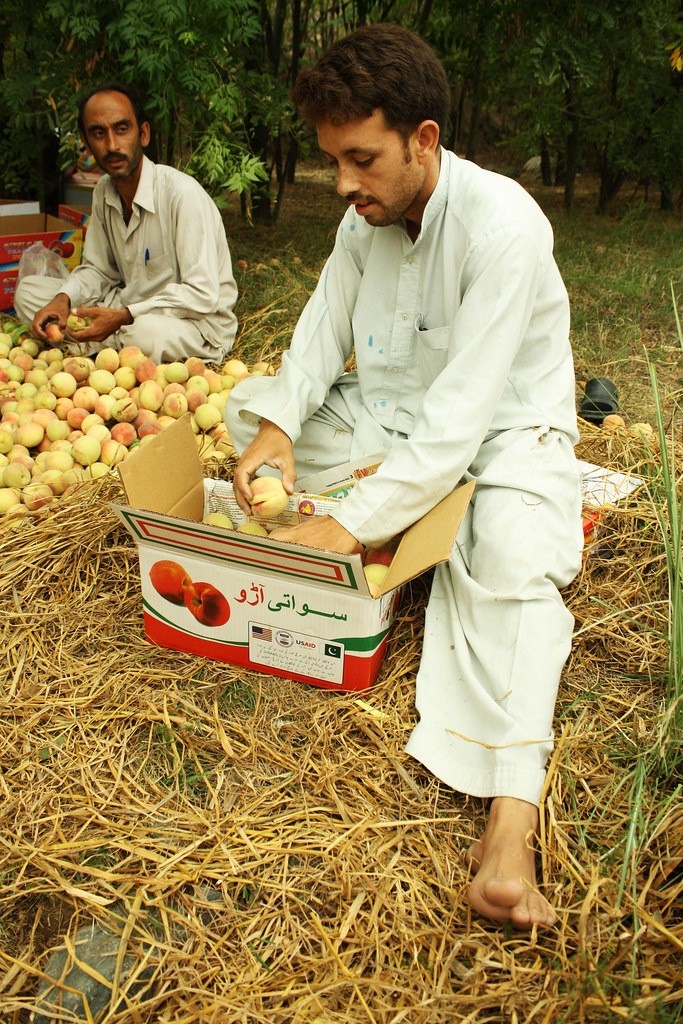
[16, 241, 70, 290]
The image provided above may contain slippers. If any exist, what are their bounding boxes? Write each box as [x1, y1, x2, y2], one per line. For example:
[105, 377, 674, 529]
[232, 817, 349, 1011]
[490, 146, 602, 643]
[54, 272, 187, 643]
[578, 377, 619, 419]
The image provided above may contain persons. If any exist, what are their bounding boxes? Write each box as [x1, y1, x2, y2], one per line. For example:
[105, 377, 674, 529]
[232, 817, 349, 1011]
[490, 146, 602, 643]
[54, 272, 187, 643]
[223, 24, 583, 928]
[14, 86, 238, 363]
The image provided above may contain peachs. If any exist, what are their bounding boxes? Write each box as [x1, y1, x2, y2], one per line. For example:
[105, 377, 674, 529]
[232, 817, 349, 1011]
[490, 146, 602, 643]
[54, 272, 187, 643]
[48, 240, 75, 258]
[150, 560, 231, 626]
[200, 476, 396, 597]
[602, 414, 653, 435]
[0, 314, 275, 519]
[238, 255, 301, 271]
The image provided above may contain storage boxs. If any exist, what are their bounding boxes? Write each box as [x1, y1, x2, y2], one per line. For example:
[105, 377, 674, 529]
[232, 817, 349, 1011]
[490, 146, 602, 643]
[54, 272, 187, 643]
[580, 460, 645, 557]
[0, 213, 84, 312]
[110, 412, 476, 696]
[58, 204, 92, 264]
[0, 199, 40, 216]
